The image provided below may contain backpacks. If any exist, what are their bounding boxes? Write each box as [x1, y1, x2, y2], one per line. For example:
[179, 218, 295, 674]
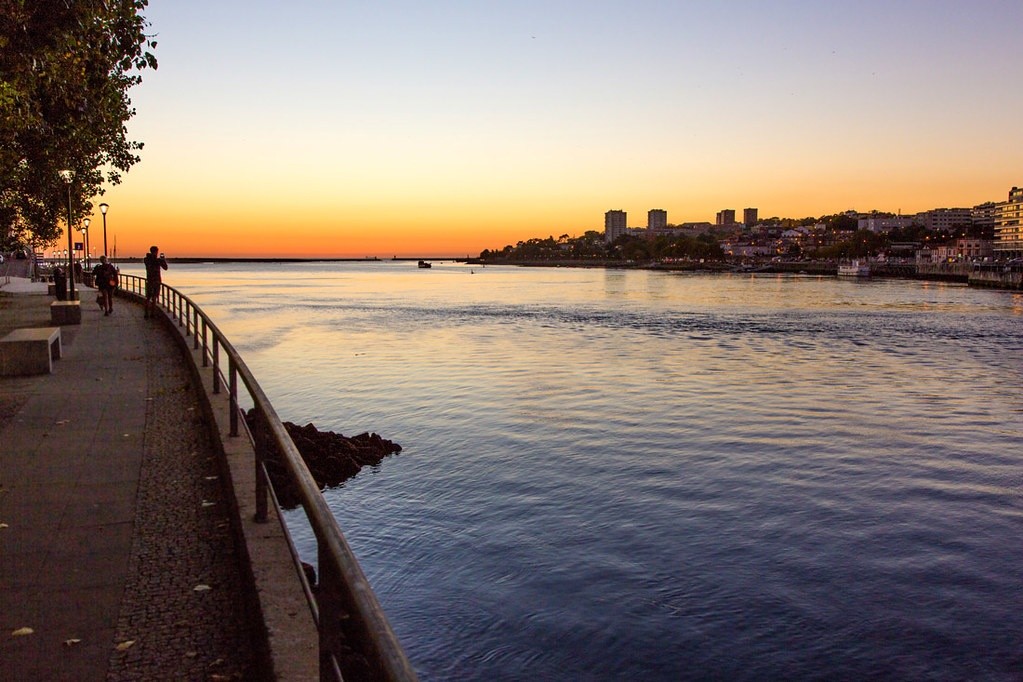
[97, 290, 105, 305]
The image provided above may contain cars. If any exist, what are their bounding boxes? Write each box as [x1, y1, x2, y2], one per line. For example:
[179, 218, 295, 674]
[0, 255, 4, 263]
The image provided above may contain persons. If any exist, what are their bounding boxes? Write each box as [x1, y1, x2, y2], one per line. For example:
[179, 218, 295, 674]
[74, 261, 82, 283]
[92, 256, 119, 316]
[144, 246, 168, 320]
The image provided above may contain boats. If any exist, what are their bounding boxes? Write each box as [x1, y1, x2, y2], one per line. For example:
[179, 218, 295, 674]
[836, 258, 871, 278]
[417, 260, 432, 269]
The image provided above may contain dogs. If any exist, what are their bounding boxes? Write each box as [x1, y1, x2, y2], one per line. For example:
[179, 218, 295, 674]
[96, 294, 105, 309]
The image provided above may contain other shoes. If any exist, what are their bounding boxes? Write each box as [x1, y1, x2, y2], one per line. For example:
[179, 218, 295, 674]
[104, 311, 109, 316]
[108, 308, 114, 313]
[152, 313, 159, 318]
[144, 313, 149, 320]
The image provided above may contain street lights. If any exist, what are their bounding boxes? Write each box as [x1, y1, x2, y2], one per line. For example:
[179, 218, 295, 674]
[83, 218, 92, 270]
[52, 250, 56, 264]
[63, 249, 68, 265]
[73, 248, 77, 264]
[58, 165, 77, 303]
[57, 250, 61, 265]
[98, 202, 110, 256]
[81, 227, 87, 270]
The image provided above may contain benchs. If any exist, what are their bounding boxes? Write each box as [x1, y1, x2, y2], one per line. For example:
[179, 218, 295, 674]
[51, 300, 81, 325]
[48, 283, 56, 296]
[0, 328, 61, 376]
[67, 288, 80, 300]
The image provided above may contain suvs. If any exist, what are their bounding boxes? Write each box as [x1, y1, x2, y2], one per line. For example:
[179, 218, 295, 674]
[16, 250, 28, 259]
